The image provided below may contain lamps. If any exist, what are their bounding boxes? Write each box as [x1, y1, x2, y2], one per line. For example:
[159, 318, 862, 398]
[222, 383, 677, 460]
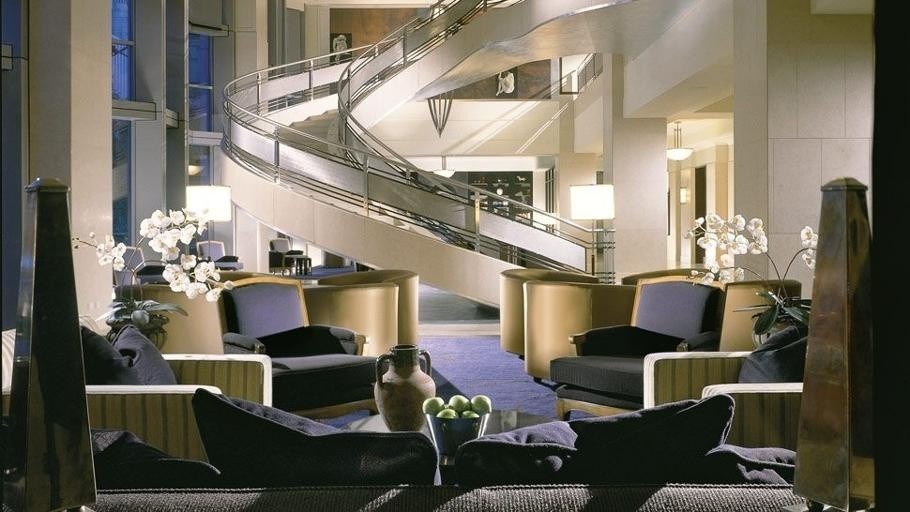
[668, 121, 692, 160]
[497, 189, 502, 195]
[570, 183, 614, 276]
[679, 187, 688, 204]
[435, 159, 453, 179]
[184, 186, 234, 258]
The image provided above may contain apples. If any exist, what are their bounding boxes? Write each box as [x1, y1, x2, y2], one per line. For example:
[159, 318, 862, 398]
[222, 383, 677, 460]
[471, 395, 491, 413]
[448, 395, 470, 415]
[422, 397, 445, 413]
[437, 409, 459, 419]
[461, 411, 478, 419]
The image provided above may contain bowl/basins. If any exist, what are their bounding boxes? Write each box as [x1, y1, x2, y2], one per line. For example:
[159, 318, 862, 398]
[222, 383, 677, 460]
[439, 454, 467, 485]
[426, 413, 491, 457]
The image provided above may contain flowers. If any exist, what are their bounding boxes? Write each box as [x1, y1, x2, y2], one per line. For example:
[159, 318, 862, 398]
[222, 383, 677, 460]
[685, 212, 820, 334]
[69, 206, 233, 326]
